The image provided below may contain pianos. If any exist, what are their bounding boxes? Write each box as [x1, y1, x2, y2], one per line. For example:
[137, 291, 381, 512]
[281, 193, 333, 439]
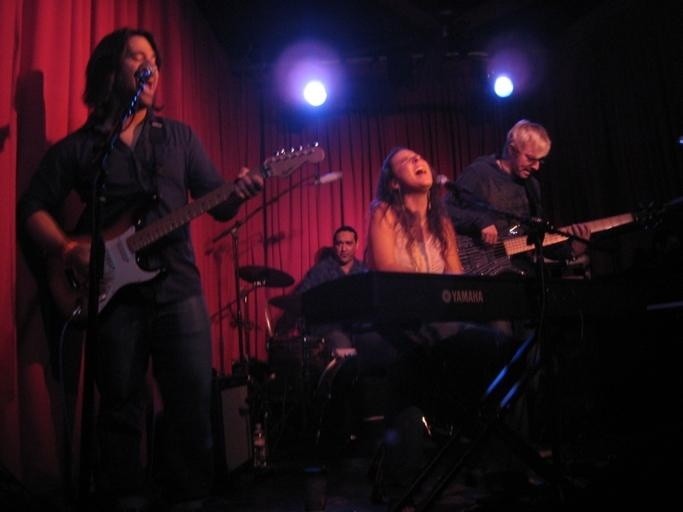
[301, 272, 584, 322]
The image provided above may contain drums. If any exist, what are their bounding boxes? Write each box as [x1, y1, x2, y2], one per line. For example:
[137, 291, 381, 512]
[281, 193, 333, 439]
[262, 337, 329, 417]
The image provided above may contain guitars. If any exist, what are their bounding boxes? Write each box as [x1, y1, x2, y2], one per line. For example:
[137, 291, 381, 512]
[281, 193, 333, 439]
[455, 202, 664, 274]
[47, 143, 325, 316]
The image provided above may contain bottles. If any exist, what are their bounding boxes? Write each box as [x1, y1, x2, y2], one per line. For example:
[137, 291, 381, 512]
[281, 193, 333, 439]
[252, 423, 268, 467]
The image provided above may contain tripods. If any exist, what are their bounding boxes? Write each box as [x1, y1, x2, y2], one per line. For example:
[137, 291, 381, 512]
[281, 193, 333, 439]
[251, 308, 329, 482]
[483, 238, 633, 512]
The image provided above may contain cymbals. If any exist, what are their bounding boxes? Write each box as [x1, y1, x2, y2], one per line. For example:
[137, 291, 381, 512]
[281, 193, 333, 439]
[268, 296, 303, 312]
[238, 266, 294, 288]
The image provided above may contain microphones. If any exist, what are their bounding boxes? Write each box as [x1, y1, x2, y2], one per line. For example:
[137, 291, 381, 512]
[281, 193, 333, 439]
[137, 60, 154, 80]
[314, 170, 343, 185]
[436, 174, 475, 197]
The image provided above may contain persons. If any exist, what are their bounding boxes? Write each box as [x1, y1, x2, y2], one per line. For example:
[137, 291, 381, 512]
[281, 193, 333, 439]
[293, 224, 370, 357]
[354, 143, 511, 511]
[17, 28, 264, 510]
[555, 237, 592, 279]
[440, 121, 590, 484]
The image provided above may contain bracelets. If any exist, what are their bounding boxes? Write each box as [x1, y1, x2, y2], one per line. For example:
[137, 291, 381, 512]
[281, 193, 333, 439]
[59, 239, 78, 258]
